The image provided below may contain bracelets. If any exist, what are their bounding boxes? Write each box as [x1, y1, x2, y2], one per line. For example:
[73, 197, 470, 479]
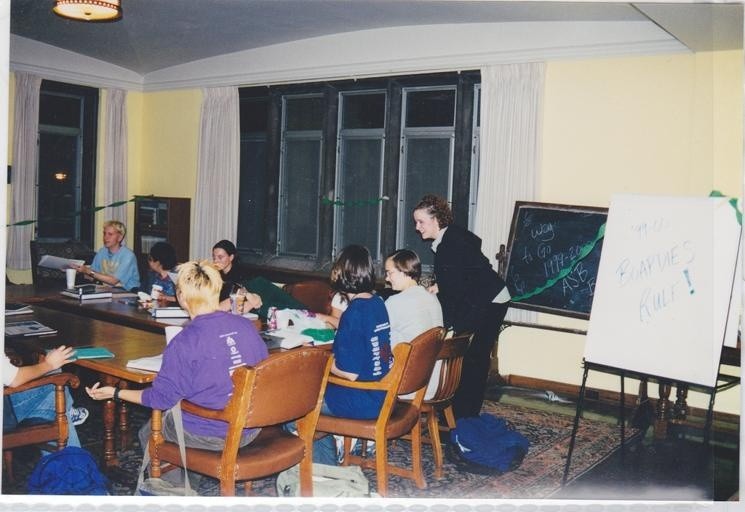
[113, 387, 120, 400]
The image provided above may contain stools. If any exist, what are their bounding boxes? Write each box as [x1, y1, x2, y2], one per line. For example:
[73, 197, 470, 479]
[2, 356, 79, 485]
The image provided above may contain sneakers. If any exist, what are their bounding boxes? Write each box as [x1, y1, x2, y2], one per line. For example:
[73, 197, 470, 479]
[70, 407, 89, 426]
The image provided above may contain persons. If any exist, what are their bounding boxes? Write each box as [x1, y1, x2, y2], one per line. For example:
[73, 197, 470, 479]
[143, 242, 178, 302]
[82, 259, 272, 484]
[282, 244, 394, 466]
[384, 247, 455, 402]
[4, 343, 91, 465]
[316, 267, 348, 331]
[67, 221, 141, 292]
[218, 275, 309, 322]
[211, 239, 248, 305]
[411, 194, 513, 416]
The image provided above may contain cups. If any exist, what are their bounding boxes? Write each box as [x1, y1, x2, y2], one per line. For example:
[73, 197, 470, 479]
[230, 294, 245, 316]
[66, 268, 75, 289]
[151, 285, 163, 300]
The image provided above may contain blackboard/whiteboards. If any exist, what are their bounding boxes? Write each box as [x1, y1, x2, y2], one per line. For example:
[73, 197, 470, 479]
[498, 200, 609, 321]
[582, 193, 743, 393]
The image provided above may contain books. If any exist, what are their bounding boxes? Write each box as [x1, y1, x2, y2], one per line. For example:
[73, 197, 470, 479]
[5, 320, 58, 338]
[38, 255, 84, 273]
[126, 324, 184, 374]
[153, 307, 191, 319]
[6, 302, 34, 316]
[243, 312, 259, 322]
[60, 287, 113, 300]
[45, 348, 114, 361]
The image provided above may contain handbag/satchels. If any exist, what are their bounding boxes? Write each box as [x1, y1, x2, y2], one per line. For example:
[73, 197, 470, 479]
[27, 446, 108, 494]
[134, 477, 198, 496]
[276, 462, 370, 496]
[446, 414, 530, 475]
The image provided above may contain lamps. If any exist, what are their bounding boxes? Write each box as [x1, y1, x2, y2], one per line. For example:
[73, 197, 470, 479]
[51, 1, 125, 24]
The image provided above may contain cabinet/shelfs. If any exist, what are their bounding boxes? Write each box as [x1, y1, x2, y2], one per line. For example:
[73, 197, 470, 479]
[134, 194, 192, 275]
